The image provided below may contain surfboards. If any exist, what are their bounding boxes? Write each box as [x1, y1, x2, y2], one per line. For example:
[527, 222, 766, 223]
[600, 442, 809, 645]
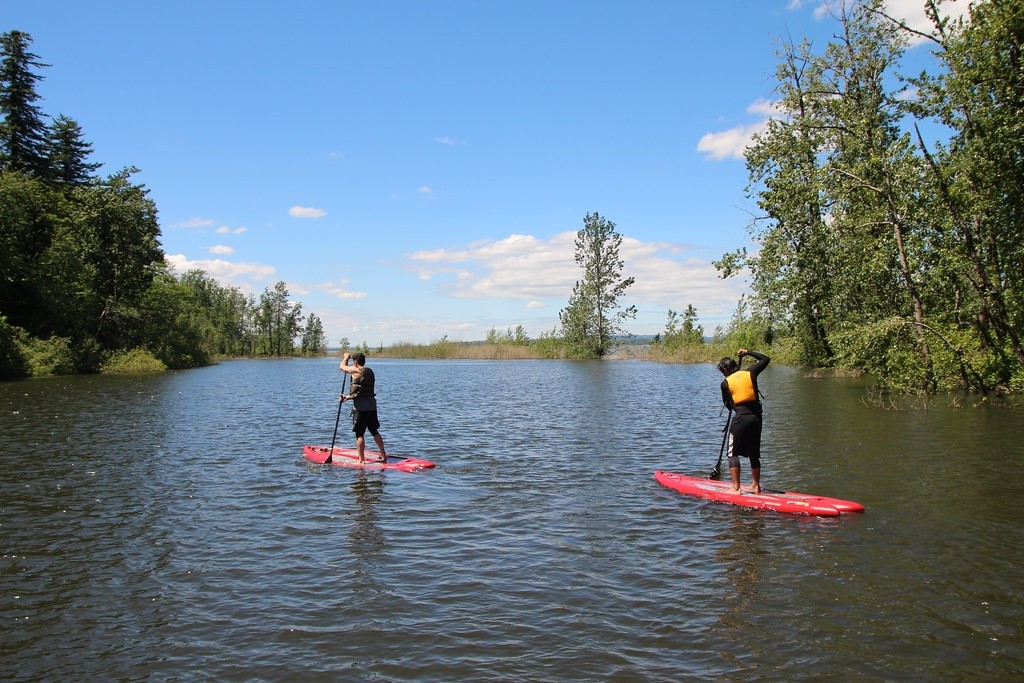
[653, 469, 867, 516]
[304, 445, 435, 472]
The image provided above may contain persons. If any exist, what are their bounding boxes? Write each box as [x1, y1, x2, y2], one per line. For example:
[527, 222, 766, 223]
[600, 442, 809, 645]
[338, 352, 388, 464]
[717, 348, 771, 495]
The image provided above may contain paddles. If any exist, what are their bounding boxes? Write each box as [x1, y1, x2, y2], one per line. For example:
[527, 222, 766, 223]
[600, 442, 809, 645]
[323, 362, 349, 465]
[710, 354, 742, 479]
